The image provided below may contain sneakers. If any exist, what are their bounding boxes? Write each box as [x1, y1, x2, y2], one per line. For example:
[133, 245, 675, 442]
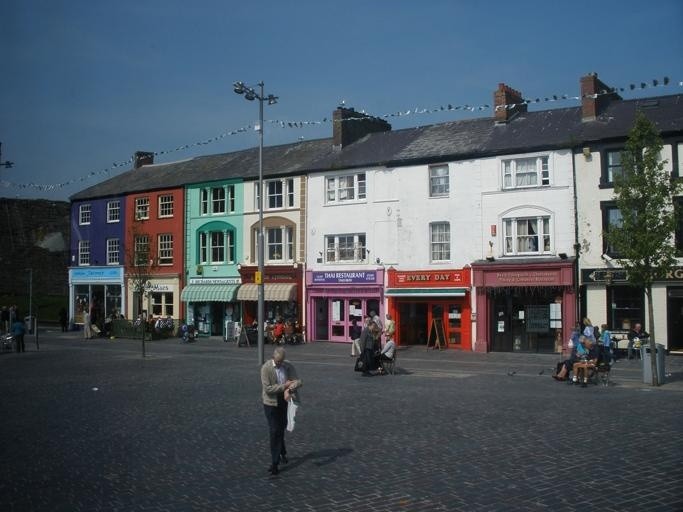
[552, 375, 588, 387]
[273, 464, 278, 475]
[282, 456, 287, 464]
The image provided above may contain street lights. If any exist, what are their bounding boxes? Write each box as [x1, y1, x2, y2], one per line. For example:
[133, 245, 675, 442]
[233, 81, 279, 365]
[25, 267, 39, 335]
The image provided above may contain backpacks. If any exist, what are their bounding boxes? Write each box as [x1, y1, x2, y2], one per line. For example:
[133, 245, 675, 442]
[389, 320, 395, 334]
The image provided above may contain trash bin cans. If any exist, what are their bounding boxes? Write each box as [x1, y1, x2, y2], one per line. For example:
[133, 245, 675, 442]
[24, 316, 36, 333]
[642, 343, 665, 384]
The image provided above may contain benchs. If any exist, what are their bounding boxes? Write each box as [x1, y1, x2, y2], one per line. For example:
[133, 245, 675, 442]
[612, 334, 640, 360]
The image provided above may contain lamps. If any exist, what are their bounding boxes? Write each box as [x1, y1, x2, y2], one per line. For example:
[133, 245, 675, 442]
[603, 269, 613, 285]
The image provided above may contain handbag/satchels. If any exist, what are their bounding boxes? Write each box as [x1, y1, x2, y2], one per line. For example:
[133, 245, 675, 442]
[355, 358, 367, 372]
[568, 339, 574, 348]
[286, 397, 298, 432]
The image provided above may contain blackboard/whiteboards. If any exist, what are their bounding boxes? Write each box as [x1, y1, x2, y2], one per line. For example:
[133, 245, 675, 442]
[434, 317, 447, 346]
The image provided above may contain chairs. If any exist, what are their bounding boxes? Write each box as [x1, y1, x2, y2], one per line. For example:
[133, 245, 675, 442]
[380, 346, 396, 375]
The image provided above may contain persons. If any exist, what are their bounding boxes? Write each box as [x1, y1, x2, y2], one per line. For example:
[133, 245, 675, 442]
[82, 310, 92, 338]
[250, 318, 302, 343]
[349, 310, 397, 377]
[259, 346, 298, 476]
[104, 314, 173, 334]
[0, 303, 26, 353]
[58, 307, 68, 333]
[553, 320, 652, 389]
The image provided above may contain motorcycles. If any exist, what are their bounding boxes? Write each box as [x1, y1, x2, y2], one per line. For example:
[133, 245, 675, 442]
[181, 324, 199, 343]
